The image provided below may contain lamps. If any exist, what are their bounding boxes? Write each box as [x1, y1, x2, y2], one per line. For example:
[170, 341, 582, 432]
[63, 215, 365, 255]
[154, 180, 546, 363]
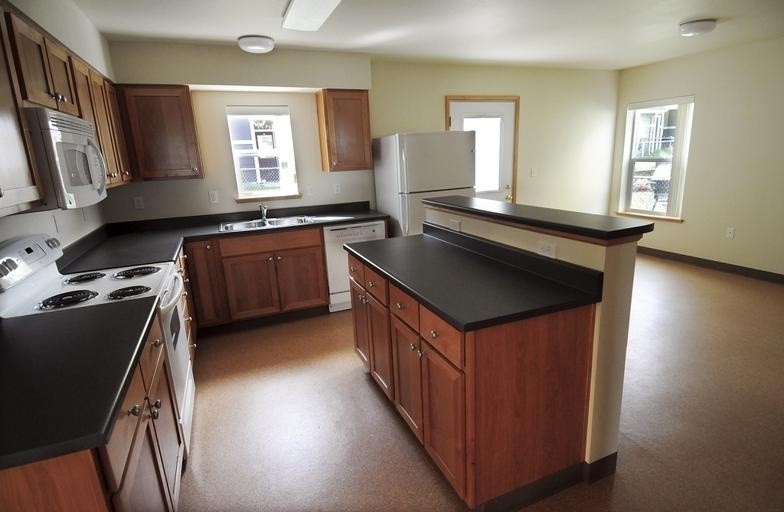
[679, 20, 715, 42]
[230, 33, 279, 57]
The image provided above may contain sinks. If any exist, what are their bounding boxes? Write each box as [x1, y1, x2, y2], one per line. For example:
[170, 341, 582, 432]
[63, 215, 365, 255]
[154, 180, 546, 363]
[218, 219, 267, 232]
[268, 215, 314, 228]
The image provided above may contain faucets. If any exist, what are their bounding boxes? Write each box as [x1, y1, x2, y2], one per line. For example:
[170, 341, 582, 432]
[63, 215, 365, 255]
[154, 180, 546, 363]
[258, 203, 268, 218]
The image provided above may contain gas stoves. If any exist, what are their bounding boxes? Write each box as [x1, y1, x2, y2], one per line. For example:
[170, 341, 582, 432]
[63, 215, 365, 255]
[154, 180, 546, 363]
[34, 262, 171, 312]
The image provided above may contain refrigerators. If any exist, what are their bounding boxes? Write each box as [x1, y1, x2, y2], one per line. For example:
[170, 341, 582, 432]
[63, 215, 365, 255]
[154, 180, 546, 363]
[372, 131, 475, 235]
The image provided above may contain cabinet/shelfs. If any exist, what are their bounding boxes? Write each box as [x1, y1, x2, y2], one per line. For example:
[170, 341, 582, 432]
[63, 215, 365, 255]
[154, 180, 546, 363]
[389, 283, 464, 502]
[314, 92, 371, 172]
[98, 314, 184, 511]
[348, 251, 389, 396]
[184, 241, 231, 328]
[11, 24, 83, 114]
[221, 225, 330, 325]
[74, 60, 136, 186]
[122, 86, 202, 178]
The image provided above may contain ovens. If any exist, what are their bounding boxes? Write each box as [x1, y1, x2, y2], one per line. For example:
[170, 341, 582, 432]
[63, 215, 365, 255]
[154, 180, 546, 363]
[157, 262, 196, 455]
[43, 108, 108, 211]
